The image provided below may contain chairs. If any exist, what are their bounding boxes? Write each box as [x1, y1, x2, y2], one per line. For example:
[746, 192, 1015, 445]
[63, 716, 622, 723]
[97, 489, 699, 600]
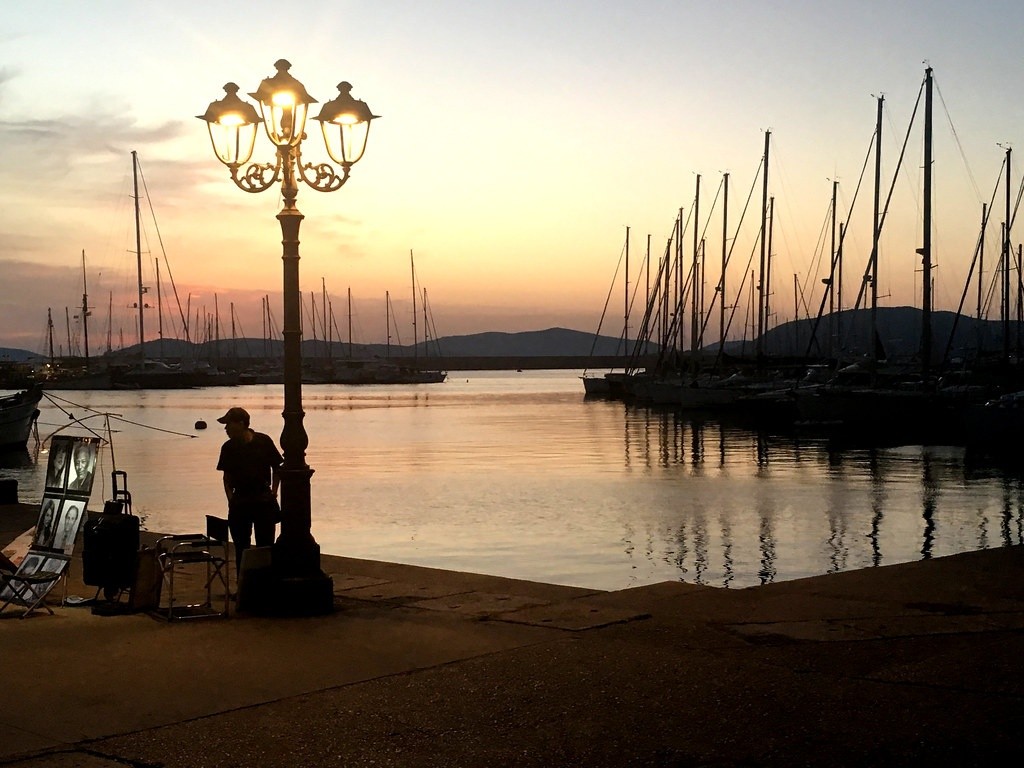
[154, 516, 229, 622]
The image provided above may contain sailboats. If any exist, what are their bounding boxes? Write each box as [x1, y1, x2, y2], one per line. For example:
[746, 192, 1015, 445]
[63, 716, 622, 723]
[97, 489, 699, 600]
[577, 66, 1023, 438]
[32, 144, 452, 391]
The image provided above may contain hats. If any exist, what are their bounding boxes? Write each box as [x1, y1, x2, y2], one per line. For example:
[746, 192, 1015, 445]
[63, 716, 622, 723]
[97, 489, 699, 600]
[217, 408, 250, 425]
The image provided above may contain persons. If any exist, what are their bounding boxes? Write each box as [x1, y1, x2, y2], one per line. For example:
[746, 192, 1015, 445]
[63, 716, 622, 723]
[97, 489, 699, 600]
[45, 442, 92, 493]
[33, 500, 79, 554]
[4, 557, 62, 602]
[216, 408, 285, 601]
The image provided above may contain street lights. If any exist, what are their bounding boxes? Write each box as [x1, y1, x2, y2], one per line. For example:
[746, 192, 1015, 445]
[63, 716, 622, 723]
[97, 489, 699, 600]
[196, 55, 378, 623]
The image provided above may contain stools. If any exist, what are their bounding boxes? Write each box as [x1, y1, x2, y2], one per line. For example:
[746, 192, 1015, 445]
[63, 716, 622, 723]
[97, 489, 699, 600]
[0, 571, 62, 620]
[91, 584, 133, 607]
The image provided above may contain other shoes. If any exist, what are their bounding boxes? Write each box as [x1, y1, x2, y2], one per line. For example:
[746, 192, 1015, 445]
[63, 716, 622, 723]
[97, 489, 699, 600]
[230, 592, 237, 601]
